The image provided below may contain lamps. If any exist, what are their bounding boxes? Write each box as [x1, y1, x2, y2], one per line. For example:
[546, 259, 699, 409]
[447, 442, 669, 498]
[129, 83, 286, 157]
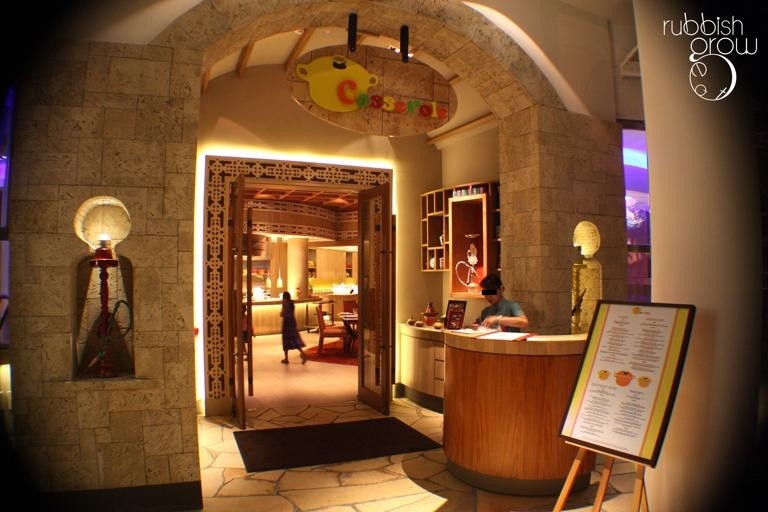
[266, 237, 272, 289]
[90, 233, 119, 378]
[276, 237, 284, 288]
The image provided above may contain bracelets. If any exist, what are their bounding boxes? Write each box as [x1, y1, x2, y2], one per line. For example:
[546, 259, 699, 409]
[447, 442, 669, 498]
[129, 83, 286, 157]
[496, 314, 504, 325]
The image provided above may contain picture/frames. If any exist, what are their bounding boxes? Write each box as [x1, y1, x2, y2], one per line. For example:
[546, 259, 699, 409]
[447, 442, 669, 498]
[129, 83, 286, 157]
[444, 301, 467, 328]
[447, 311, 463, 330]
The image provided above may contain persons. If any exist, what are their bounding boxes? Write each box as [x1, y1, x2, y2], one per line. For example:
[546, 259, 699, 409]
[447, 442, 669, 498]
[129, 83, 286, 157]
[279, 291, 309, 365]
[237, 303, 257, 360]
[469, 274, 530, 332]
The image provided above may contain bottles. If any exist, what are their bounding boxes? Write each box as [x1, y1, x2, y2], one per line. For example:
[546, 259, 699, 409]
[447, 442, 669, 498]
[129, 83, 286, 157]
[424, 301, 435, 325]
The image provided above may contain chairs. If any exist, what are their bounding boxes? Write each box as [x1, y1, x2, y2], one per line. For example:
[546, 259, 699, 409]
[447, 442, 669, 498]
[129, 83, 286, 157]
[316, 300, 359, 356]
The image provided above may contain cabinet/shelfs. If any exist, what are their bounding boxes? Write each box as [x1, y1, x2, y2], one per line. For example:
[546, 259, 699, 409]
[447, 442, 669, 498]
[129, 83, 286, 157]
[419, 180, 501, 298]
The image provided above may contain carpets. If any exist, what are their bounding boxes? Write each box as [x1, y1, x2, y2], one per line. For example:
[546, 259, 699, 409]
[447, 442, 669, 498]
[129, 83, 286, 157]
[233, 417, 443, 473]
[299, 335, 359, 366]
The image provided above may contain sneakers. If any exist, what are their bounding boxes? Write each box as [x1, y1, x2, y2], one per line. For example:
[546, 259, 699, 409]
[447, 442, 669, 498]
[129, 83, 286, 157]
[281, 359, 289, 364]
[302, 357, 308, 364]
